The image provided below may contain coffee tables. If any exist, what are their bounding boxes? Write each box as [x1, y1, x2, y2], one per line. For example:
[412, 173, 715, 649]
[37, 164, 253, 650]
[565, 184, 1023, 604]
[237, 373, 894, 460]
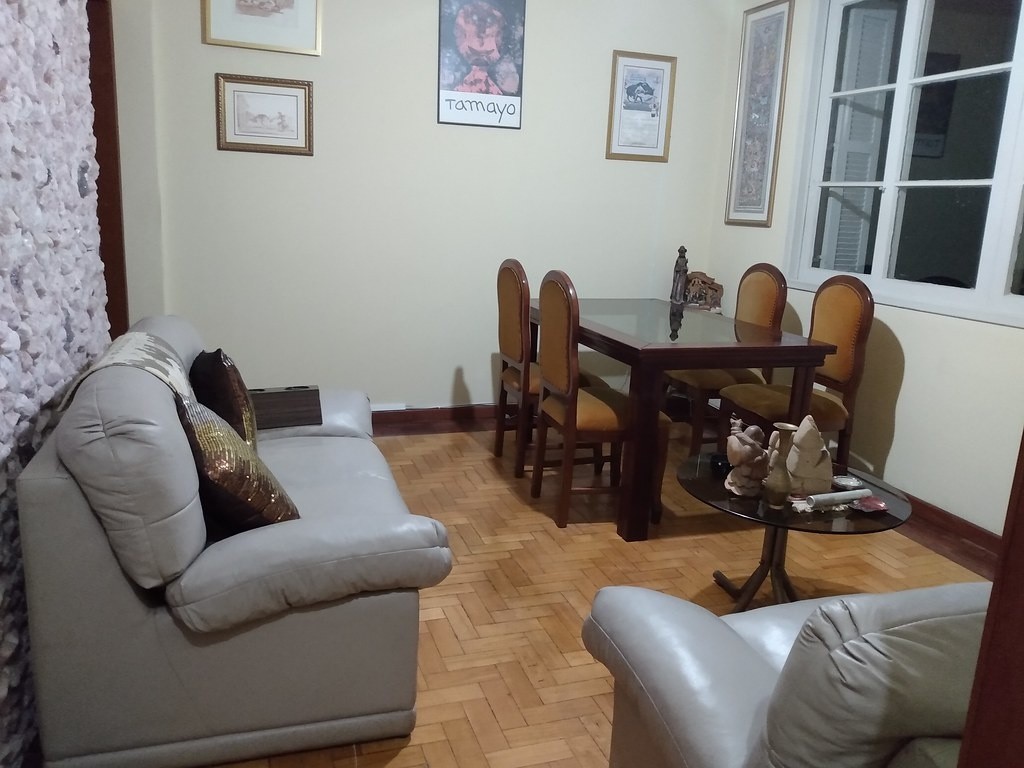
[676, 453, 912, 614]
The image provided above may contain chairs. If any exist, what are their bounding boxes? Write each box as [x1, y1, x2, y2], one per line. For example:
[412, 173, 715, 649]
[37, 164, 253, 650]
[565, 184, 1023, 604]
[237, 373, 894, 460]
[660, 262, 787, 457]
[532, 269, 673, 528]
[493, 258, 602, 479]
[717, 275, 874, 463]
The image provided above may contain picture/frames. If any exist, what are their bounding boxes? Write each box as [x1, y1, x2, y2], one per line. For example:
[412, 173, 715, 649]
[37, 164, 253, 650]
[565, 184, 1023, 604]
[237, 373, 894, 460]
[215, 72, 313, 156]
[724, 0, 796, 227]
[200, 0, 322, 57]
[603, 50, 678, 163]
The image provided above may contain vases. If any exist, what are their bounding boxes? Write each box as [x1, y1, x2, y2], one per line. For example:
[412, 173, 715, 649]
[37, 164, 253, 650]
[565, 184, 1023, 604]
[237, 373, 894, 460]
[766, 422, 798, 502]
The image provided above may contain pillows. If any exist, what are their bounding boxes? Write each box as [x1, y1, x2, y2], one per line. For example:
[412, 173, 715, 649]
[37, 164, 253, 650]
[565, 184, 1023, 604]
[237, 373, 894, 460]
[174, 389, 300, 532]
[190, 347, 257, 453]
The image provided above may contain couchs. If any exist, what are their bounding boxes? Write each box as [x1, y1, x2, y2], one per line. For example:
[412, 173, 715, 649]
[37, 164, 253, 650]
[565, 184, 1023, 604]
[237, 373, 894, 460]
[17, 314, 452, 768]
[582, 582, 994, 767]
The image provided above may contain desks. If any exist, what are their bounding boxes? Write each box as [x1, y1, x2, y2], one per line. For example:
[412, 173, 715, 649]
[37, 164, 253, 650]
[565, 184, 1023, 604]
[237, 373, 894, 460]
[529, 298, 838, 543]
